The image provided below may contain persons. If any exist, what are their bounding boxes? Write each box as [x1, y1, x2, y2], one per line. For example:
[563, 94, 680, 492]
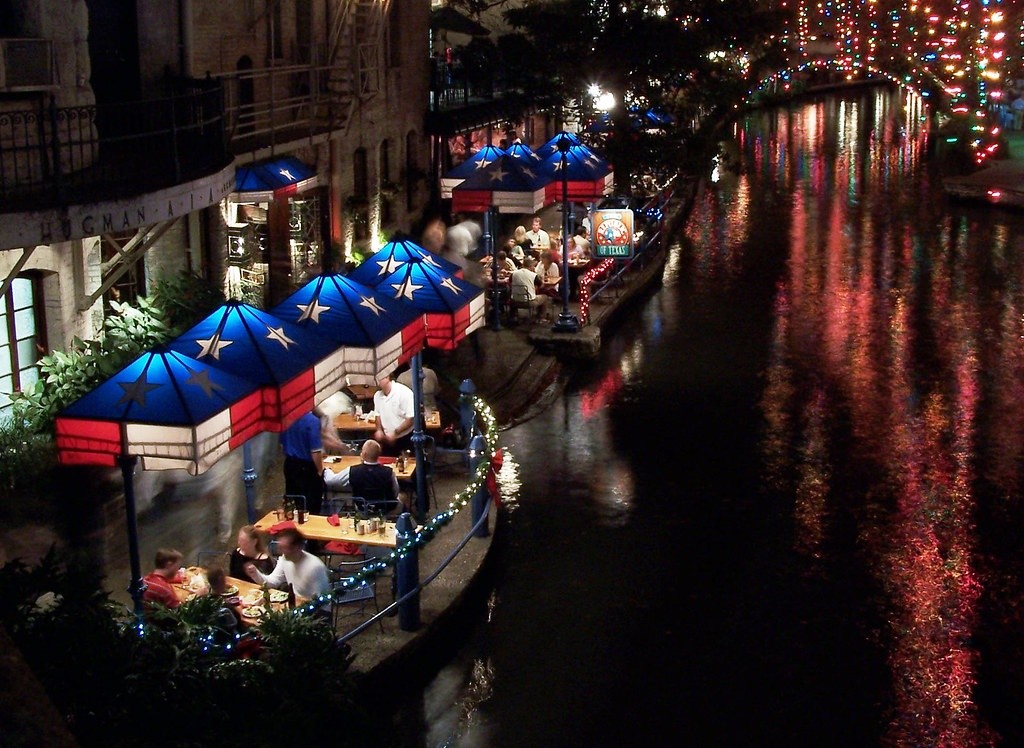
[143, 523, 332, 641]
[282, 356, 438, 519]
[493, 217, 593, 325]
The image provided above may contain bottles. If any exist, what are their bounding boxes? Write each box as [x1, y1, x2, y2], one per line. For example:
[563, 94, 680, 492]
[341, 511, 385, 535]
[278, 498, 309, 524]
[395, 457, 409, 472]
[537, 235, 541, 246]
[544, 269, 548, 282]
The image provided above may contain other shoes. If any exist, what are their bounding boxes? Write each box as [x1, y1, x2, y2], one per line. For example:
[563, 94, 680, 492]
[512, 317, 518, 324]
[536, 319, 550, 325]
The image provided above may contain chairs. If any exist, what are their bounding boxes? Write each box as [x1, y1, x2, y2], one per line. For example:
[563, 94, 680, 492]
[410, 436, 437, 509]
[366, 500, 398, 522]
[270, 541, 307, 558]
[327, 496, 365, 519]
[504, 282, 540, 320]
[329, 557, 383, 634]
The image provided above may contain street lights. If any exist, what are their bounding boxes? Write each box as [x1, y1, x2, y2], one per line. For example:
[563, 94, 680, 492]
[555, 133, 578, 331]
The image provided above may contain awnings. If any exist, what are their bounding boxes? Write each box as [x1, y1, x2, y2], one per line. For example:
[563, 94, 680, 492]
[229, 153, 317, 203]
[580, 103, 672, 146]
[440, 132, 614, 231]
[52, 232, 486, 475]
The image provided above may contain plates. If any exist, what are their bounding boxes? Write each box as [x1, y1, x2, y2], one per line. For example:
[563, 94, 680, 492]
[189, 574, 290, 618]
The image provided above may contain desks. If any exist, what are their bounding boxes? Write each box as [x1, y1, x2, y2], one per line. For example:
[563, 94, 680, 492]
[169, 567, 289, 628]
[522, 255, 590, 301]
[253, 510, 423, 566]
[334, 410, 441, 432]
[320, 455, 417, 508]
[485, 272, 563, 318]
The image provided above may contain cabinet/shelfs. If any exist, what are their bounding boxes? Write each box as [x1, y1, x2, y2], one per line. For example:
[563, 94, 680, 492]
[347, 377, 394, 400]
